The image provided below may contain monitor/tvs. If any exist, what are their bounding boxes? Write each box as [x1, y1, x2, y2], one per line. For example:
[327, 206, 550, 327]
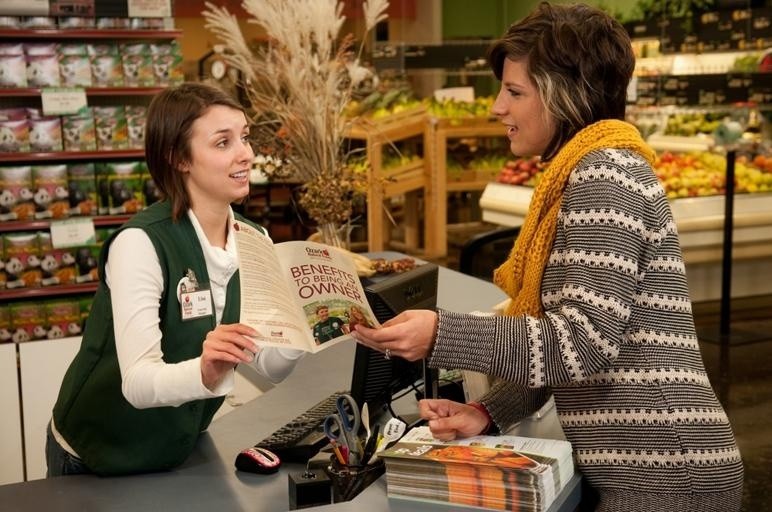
[352, 263, 439, 433]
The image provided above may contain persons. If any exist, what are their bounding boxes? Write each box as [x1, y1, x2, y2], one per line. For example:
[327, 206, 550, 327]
[312, 305, 349, 346]
[348, 304, 372, 333]
[44, 81, 305, 478]
[347, 0, 747, 512]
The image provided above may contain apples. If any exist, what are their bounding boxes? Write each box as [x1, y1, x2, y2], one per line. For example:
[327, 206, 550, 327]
[496, 157, 550, 186]
[653, 147, 771, 198]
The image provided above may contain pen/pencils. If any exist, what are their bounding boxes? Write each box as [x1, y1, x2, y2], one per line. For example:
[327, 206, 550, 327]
[330, 425, 385, 466]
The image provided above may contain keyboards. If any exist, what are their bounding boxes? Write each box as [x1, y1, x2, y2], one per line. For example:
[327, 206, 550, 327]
[256, 391, 386, 454]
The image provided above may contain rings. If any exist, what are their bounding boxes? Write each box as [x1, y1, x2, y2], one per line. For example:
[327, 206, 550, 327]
[384, 348, 392, 360]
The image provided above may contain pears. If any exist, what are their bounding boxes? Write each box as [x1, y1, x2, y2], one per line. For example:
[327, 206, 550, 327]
[428, 95, 490, 118]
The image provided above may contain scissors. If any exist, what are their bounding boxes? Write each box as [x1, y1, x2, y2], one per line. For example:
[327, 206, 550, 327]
[324, 394, 360, 464]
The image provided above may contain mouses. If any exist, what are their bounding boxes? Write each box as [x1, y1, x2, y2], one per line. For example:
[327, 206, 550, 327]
[235, 447, 280, 475]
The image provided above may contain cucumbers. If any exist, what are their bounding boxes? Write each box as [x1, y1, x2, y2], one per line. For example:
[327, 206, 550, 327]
[349, 85, 426, 119]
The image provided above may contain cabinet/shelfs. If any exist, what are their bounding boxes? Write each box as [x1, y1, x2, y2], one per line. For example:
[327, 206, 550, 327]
[336, 101, 434, 263]
[430, 117, 512, 259]
[0, 29, 184, 344]
